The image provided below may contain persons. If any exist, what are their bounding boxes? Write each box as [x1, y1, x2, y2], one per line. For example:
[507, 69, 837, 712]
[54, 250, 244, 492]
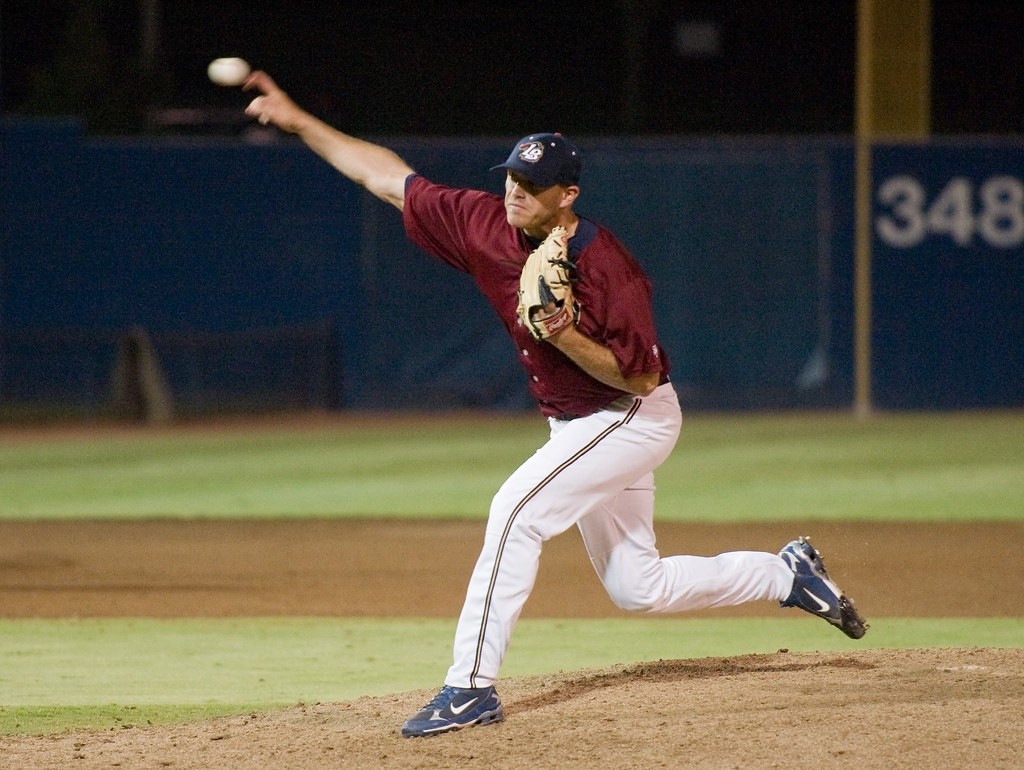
[243, 71, 871, 738]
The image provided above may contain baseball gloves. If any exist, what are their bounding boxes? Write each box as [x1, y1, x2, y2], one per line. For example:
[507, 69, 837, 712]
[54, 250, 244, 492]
[517, 227, 582, 343]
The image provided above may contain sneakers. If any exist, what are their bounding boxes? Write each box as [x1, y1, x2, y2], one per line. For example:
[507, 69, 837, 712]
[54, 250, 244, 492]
[776, 535, 871, 640]
[401, 684, 503, 738]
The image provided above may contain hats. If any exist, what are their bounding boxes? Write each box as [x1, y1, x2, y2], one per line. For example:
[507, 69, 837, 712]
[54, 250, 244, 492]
[488, 132, 588, 187]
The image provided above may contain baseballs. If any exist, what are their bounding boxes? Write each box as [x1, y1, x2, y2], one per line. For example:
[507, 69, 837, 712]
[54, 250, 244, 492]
[211, 57, 250, 85]
